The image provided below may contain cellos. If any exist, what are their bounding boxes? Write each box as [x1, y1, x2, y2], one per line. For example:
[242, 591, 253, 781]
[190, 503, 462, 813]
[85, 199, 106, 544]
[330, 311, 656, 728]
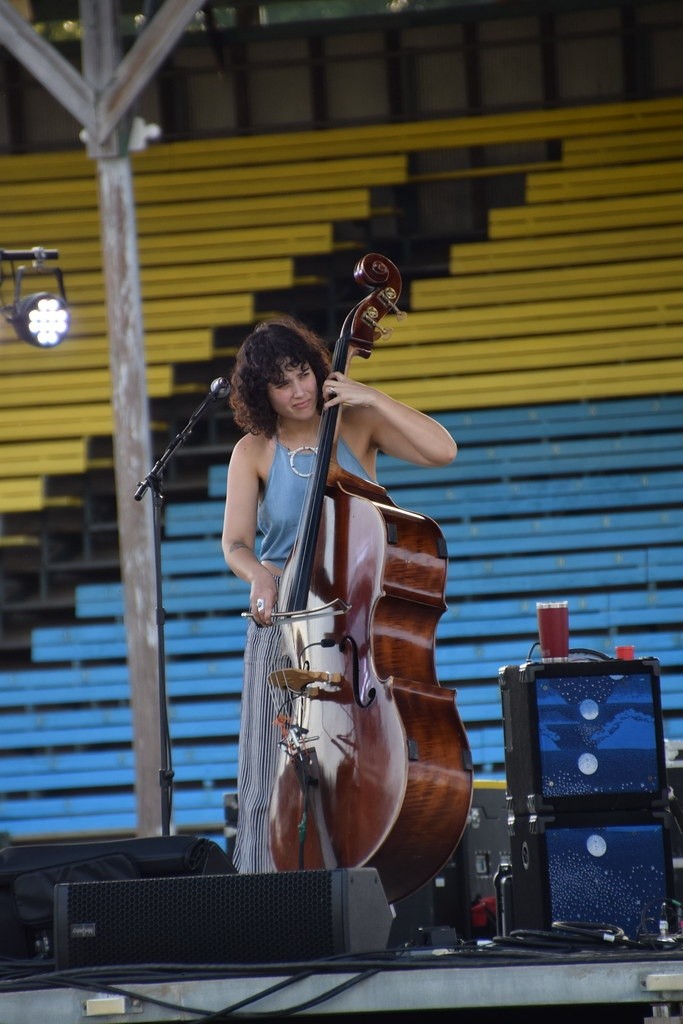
[230, 254, 476, 900]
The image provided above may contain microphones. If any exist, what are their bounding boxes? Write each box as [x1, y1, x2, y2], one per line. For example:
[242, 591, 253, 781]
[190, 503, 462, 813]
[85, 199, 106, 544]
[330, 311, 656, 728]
[209, 377, 231, 397]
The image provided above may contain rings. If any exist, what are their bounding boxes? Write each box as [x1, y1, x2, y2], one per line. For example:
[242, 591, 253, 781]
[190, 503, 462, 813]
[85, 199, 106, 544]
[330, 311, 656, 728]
[330, 386, 337, 394]
[256, 599, 265, 613]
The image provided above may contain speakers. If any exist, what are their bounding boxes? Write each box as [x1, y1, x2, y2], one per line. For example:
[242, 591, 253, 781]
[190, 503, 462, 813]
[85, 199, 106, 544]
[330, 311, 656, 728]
[1, 835, 235, 959]
[53, 864, 396, 965]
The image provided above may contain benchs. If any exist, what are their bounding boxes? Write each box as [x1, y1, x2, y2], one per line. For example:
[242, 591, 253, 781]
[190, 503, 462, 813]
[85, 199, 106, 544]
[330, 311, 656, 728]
[0, 91, 683, 832]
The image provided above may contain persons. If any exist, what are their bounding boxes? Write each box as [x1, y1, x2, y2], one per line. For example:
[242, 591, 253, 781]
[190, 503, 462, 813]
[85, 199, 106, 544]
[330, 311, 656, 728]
[206, 316, 458, 886]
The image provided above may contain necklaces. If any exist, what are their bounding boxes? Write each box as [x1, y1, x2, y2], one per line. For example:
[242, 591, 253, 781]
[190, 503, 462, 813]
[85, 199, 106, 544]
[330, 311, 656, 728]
[274, 408, 325, 479]
